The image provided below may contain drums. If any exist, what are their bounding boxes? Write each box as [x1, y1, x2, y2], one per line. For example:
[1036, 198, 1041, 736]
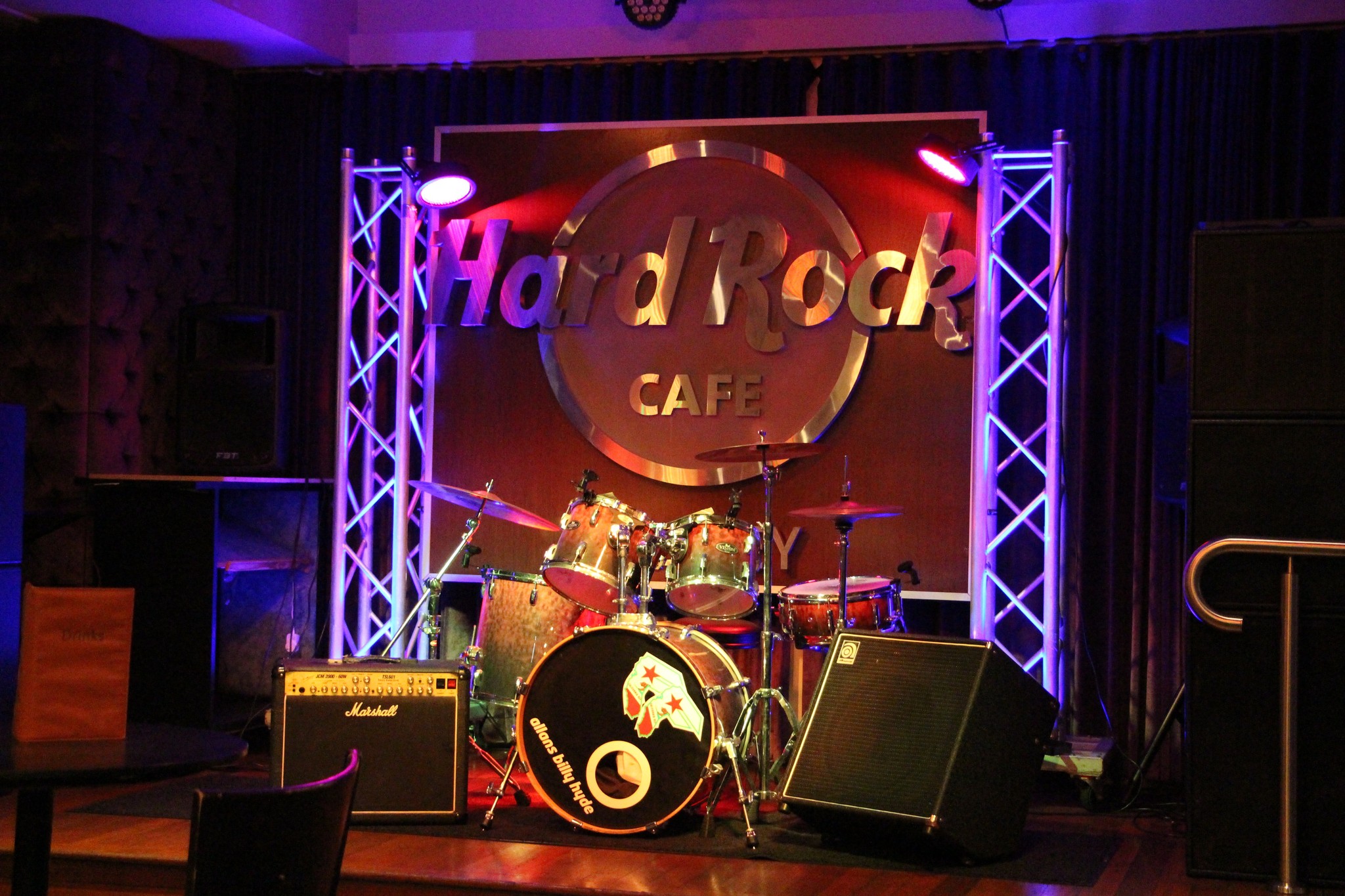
[780, 632, 837, 739]
[778, 575, 903, 636]
[542, 495, 666, 618]
[468, 565, 585, 712]
[673, 616, 789, 766]
[666, 513, 766, 619]
[516, 620, 751, 832]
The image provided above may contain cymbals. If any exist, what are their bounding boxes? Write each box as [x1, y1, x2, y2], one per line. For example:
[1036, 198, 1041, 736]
[408, 472, 561, 537]
[695, 442, 827, 464]
[790, 501, 906, 521]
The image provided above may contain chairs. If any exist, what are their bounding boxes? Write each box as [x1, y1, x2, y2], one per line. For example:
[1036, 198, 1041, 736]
[183, 747, 362, 896]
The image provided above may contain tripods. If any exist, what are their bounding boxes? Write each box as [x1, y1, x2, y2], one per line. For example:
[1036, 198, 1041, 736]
[696, 448, 800, 839]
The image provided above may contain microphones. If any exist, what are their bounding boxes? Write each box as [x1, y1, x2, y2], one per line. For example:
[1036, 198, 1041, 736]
[466, 546, 481, 555]
[582, 470, 599, 482]
[898, 561, 914, 573]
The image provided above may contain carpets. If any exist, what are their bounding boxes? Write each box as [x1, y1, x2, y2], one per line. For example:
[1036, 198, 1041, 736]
[63, 774, 1124, 889]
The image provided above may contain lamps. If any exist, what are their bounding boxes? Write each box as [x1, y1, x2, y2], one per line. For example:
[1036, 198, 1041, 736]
[614, 0, 686, 30]
[399, 157, 477, 210]
[915, 133, 1005, 187]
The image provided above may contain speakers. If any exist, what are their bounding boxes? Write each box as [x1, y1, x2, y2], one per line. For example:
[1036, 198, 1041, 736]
[182, 304, 289, 475]
[270, 659, 469, 826]
[778, 630, 1061, 863]
[1180, 220, 1345, 889]
